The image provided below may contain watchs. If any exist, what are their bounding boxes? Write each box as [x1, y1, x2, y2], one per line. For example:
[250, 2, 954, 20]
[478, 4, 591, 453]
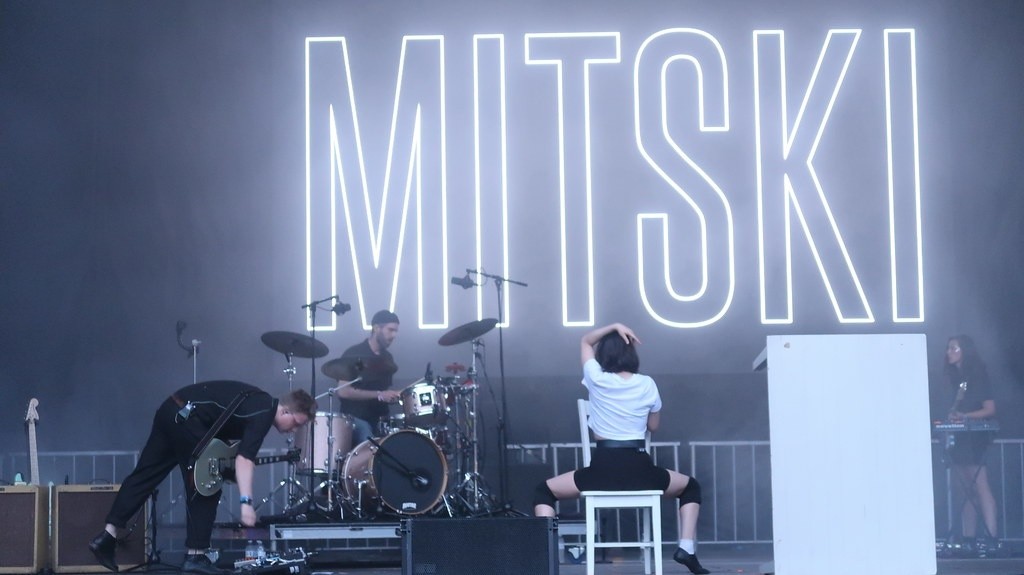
[336, 309, 400, 449]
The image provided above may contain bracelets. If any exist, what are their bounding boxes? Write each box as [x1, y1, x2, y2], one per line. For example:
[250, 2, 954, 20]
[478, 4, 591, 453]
[239, 495, 253, 506]
[377, 390, 384, 402]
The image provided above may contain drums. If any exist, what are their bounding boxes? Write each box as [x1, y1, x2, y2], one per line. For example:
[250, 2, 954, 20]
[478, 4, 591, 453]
[342, 428, 448, 514]
[295, 410, 352, 475]
[377, 412, 449, 450]
[400, 383, 449, 427]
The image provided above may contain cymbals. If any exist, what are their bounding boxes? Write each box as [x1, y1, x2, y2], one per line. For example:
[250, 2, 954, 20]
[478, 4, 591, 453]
[321, 357, 374, 381]
[438, 318, 500, 345]
[261, 331, 328, 358]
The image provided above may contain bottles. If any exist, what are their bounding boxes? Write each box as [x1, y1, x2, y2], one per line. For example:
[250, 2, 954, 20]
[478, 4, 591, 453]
[245, 540, 265, 560]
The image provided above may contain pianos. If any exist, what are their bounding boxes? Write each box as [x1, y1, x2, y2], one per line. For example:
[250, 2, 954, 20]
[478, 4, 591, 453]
[929, 416, 1006, 557]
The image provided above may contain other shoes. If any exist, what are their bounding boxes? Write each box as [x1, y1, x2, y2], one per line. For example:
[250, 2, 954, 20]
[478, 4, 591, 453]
[180, 553, 233, 575]
[88, 530, 119, 572]
[673, 547, 710, 574]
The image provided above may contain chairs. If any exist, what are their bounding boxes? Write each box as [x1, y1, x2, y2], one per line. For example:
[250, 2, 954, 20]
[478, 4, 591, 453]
[578, 398, 664, 575]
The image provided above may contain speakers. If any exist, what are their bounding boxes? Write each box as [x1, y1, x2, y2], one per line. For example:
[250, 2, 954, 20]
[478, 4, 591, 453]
[51, 483, 148, 574]
[401, 515, 560, 575]
[0, 484, 49, 575]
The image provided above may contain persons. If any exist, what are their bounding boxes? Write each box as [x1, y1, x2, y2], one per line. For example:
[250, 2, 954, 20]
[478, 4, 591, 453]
[88, 380, 316, 575]
[533, 322, 710, 575]
[946, 335, 999, 558]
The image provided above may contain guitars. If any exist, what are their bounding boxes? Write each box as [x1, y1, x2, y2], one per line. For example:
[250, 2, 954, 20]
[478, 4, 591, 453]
[14, 398, 53, 542]
[194, 436, 301, 498]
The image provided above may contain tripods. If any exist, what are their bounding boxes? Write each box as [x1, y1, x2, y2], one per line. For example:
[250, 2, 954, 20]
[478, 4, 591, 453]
[255, 344, 363, 523]
[123, 489, 182, 574]
[434, 331, 530, 518]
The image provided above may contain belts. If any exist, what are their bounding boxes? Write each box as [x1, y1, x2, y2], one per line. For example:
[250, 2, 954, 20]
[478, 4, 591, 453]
[597, 439, 645, 448]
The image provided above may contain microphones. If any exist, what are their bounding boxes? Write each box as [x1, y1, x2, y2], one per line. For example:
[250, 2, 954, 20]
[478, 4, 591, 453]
[425, 363, 431, 380]
[475, 339, 480, 357]
[332, 304, 351, 312]
[450, 278, 478, 286]
[414, 475, 431, 488]
